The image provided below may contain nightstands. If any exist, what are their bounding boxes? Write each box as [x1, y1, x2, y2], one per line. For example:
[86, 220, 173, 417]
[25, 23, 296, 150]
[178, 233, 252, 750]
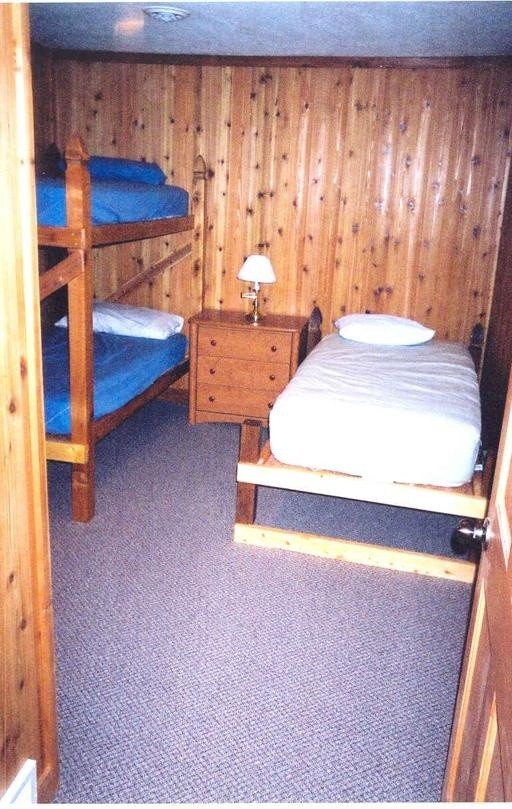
[185, 306, 311, 428]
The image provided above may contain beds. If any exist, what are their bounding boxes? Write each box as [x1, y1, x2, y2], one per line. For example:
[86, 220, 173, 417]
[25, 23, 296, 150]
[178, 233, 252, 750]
[230, 301, 491, 589]
[34, 129, 197, 523]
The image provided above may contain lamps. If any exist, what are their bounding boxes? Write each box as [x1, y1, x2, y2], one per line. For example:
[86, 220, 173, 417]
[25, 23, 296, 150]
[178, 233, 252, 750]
[236, 254, 278, 323]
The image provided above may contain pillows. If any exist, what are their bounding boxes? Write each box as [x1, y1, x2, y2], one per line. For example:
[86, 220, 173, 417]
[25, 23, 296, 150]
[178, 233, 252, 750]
[55, 150, 166, 186]
[332, 311, 436, 346]
[56, 299, 182, 341]
